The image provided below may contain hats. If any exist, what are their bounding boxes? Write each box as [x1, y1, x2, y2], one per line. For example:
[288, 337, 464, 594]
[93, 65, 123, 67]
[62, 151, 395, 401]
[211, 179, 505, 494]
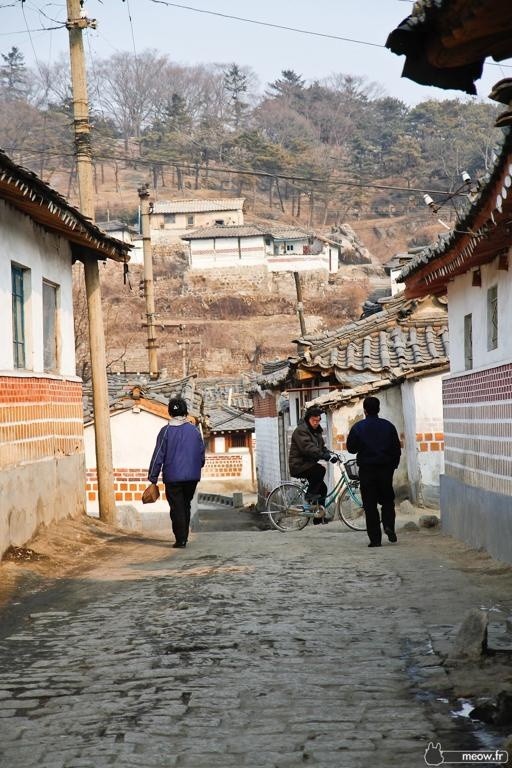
[306, 404, 326, 415]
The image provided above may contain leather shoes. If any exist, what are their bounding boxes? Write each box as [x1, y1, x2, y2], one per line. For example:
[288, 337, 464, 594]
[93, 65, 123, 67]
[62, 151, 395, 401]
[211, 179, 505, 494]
[173, 539, 186, 547]
[368, 542, 381, 547]
[305, 493, 321, 500]
[313, 517, 332, 525]
[385, 527, 397, 542]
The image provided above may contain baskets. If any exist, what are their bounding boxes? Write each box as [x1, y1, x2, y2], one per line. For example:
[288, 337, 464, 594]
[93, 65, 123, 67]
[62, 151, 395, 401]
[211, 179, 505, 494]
[344, 460, 360, 480]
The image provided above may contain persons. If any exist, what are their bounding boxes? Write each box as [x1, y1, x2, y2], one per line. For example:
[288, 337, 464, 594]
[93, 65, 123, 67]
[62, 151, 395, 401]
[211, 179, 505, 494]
[289, 407, 338, 524]
[149, 397, 204, 548]
[347, 397, 401, 547]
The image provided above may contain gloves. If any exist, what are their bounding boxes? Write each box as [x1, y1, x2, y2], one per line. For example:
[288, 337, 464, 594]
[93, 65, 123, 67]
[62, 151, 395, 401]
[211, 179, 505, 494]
[322, 453, 330, 461]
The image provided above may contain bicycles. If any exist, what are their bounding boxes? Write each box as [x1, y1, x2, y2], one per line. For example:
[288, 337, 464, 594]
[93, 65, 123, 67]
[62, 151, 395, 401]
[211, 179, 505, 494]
[262, 448, 383, 533]
